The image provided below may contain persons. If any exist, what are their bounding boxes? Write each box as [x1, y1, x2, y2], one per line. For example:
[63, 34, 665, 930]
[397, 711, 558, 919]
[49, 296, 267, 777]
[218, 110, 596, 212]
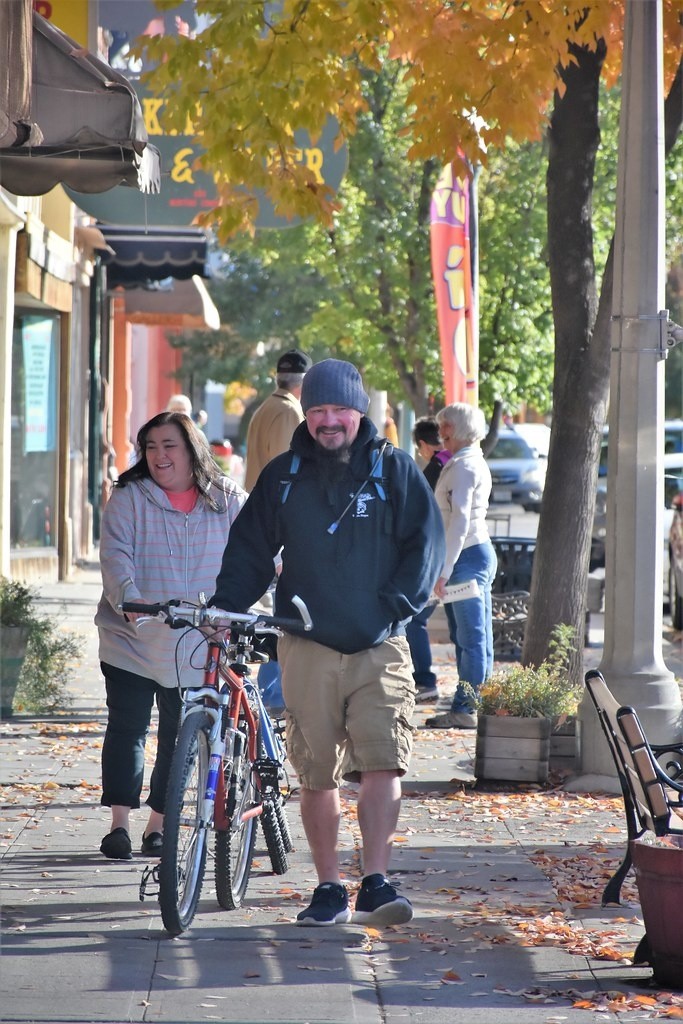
[243, 349, 313, 615]
[166, 393, 209, 447]
[201, 358, 447, 928]
[94, 412, 283, 859]
[102, 441, 117, 492]
[385, 401, 398, 448]
[425, 402, 498, 730]
[404, 418, 463, 706]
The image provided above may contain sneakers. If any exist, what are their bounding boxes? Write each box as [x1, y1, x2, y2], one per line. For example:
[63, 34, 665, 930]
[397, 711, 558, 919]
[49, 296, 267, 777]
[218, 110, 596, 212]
[295, 882, 352, 926]
[141, 831, 163, 856]
[100, 827, 132, 859]
[351, 873, 413, 925]
[426, 711, 478, 729]
[414, 684, 439, 704]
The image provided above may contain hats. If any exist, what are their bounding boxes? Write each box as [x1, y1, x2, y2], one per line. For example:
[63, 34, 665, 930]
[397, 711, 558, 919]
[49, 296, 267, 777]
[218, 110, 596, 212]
[277, 349, 313, 374]
[300, 358, 370, 414]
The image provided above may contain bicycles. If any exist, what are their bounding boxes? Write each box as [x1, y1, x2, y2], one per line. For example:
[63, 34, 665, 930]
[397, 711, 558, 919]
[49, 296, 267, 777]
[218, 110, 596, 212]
[123, 591, 314, 937]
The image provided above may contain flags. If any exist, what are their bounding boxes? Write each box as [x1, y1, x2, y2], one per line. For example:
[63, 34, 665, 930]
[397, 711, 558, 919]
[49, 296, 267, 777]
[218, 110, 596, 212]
[429, 143, 479, 410]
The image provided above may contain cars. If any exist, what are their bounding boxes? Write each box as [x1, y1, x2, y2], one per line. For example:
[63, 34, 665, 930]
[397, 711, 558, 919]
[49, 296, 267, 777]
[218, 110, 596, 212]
[481, 418, 683, 630]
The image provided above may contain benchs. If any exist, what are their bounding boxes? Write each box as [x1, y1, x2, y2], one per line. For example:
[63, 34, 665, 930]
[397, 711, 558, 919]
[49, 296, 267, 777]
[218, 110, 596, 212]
[586, 669, 683, 966]
[492, 591, 530, 653]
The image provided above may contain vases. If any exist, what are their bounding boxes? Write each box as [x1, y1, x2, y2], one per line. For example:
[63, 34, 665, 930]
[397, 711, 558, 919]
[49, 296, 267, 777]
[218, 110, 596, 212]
[630, 833, 683, 989]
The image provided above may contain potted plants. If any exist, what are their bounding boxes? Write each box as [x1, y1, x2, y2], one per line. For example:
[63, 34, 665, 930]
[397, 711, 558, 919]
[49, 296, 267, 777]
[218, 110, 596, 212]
[0, 576, 89, 719]
[458, 623, 584, 782]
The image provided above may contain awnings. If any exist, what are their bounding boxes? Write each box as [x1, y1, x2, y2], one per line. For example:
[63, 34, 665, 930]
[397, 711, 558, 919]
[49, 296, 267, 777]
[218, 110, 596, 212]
[88, 224, 212, 292]
[0, 9, 161, 197]
[125, 274, 220, 332]
